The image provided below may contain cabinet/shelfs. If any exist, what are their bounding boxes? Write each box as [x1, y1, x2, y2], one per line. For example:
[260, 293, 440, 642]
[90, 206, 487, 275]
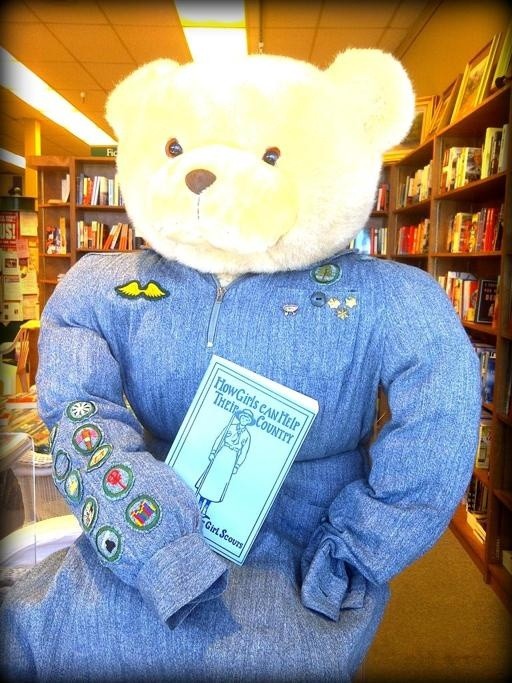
[25, 152, 151, 320]
[349, 84, 510, 610]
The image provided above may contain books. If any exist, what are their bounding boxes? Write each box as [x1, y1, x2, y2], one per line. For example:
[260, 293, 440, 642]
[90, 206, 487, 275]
[394, 160, 432, 270]
[438, 124, 510, 193]
[5, 397, 37, 409]
[57, 174, 142, 282]
[0, 413, 11, 426]
[437, 204, 504, 330]
[352, 184, 389, 254]
[462, 475, 488, 544]
[476, 410, 493, 469]
[164, 354, 319, 567]
[471, 342, 496, 402]
[495, 524, 512, 575]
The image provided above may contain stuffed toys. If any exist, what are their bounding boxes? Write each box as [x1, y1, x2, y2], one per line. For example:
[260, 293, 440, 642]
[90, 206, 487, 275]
[0, 47, 483, 683]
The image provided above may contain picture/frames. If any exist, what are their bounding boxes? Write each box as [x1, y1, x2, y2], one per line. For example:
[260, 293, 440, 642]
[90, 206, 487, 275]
[421, 30, 501, 141]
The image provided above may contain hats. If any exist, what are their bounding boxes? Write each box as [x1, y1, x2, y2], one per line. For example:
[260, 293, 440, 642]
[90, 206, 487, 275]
[235, 409, 256, 428]
